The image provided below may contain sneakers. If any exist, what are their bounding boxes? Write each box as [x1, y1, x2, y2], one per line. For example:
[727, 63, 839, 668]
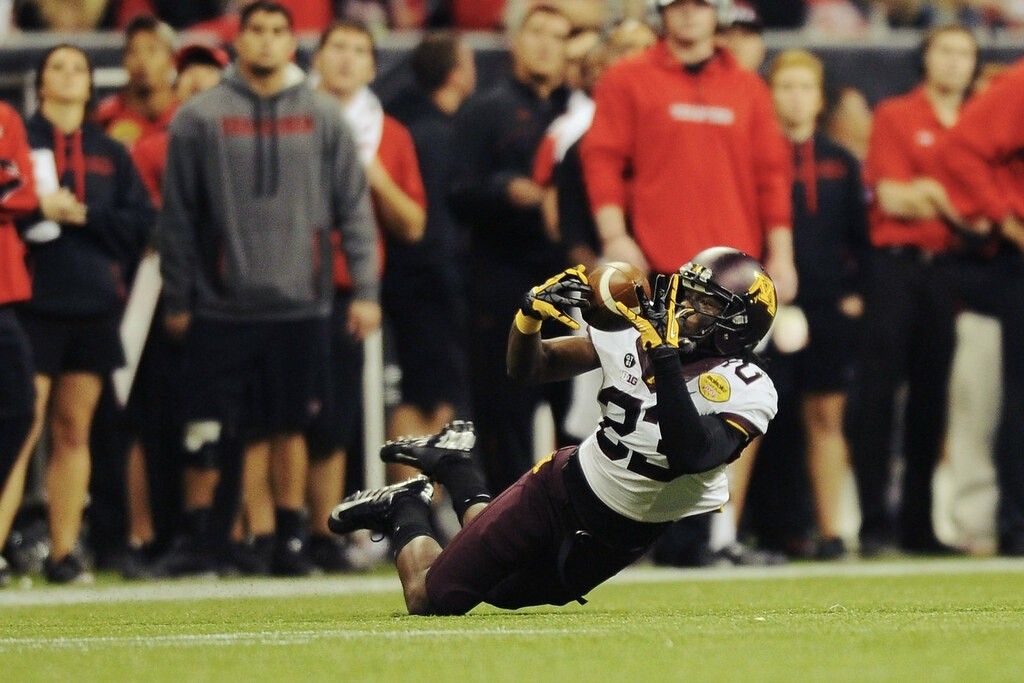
[328, 474, 435, 543]
[380, 420, 478, 476]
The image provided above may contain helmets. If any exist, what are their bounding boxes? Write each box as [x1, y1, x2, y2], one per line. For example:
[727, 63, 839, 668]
[668, 246, 778, 357]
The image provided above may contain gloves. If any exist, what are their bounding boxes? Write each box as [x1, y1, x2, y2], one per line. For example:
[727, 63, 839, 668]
[614, 271, 679, 369]
[514, 264, 592, 336]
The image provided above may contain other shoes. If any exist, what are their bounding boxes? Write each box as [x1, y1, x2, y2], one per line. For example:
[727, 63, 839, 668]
[1, 531, 378, 586]
[901, 532, 968, 556]
[820, 534, 863, 564]
[699, 540, 787, 570]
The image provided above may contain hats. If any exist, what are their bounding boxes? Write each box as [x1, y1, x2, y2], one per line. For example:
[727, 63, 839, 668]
[719, 8, 762, 31]
[175, 42, 226, 70]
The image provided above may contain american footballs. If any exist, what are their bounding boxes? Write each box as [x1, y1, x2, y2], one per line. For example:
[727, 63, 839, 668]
[580, 262, 653, 332]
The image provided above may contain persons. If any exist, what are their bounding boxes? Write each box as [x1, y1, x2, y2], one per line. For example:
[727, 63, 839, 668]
[584, 0, 800, 568]
[0, 44, 152, 582]
[162, 0, 381, 578]
[709, 0, 1024, 561]
[274, 1, 666, 568]
[0, 102, 37, 496]
[327, 246, 777, 616]
[11, 0, 247, 574]
